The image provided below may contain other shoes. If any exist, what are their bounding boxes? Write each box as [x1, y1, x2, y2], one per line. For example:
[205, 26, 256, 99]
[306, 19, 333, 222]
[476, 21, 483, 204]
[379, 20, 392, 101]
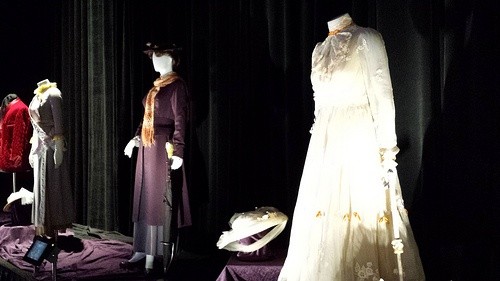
[119, 256, 146, 267]
[144, 266, 154, 278]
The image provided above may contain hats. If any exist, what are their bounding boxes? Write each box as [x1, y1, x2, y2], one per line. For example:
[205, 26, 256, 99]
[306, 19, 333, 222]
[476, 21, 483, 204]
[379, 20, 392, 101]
[3, 186, 33, 212]
[216, 206, 288, 253]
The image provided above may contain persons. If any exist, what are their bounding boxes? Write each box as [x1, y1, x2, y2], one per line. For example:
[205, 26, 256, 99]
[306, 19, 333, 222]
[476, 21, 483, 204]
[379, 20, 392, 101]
[277, 13, 425, 281]
[0, 79, 75, 237]
[119, 40, 192, 275]
